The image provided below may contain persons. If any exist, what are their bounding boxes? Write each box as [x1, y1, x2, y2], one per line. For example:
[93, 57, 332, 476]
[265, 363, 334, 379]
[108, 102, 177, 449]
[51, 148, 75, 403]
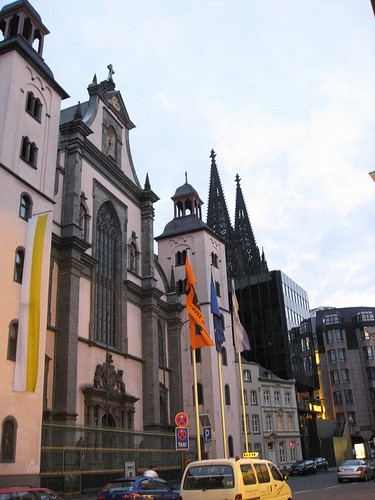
[144, 464, 158, 477]
[75, 436, 84, 466]
[138, 440, 147, 462]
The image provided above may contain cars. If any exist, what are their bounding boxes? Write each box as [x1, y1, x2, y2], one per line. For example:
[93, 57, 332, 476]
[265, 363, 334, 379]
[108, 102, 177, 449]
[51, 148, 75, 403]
[96, 477, 179, 500]
[337, 460, 373, 483]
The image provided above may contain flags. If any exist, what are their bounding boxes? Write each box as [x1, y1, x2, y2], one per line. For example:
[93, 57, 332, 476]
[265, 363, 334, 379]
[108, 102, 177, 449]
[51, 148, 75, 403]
[231, 277, 252, 354]
[186, 254, 215, 350]
[209, 269, 226, 355]
[12, 211, 54, 394]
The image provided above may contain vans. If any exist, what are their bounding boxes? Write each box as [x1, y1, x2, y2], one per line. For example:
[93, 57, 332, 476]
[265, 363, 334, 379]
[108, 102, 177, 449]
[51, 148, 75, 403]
[308, 457, 328, 471]
[179, 458, 293, 500]
[289, 459, 316, 476]
